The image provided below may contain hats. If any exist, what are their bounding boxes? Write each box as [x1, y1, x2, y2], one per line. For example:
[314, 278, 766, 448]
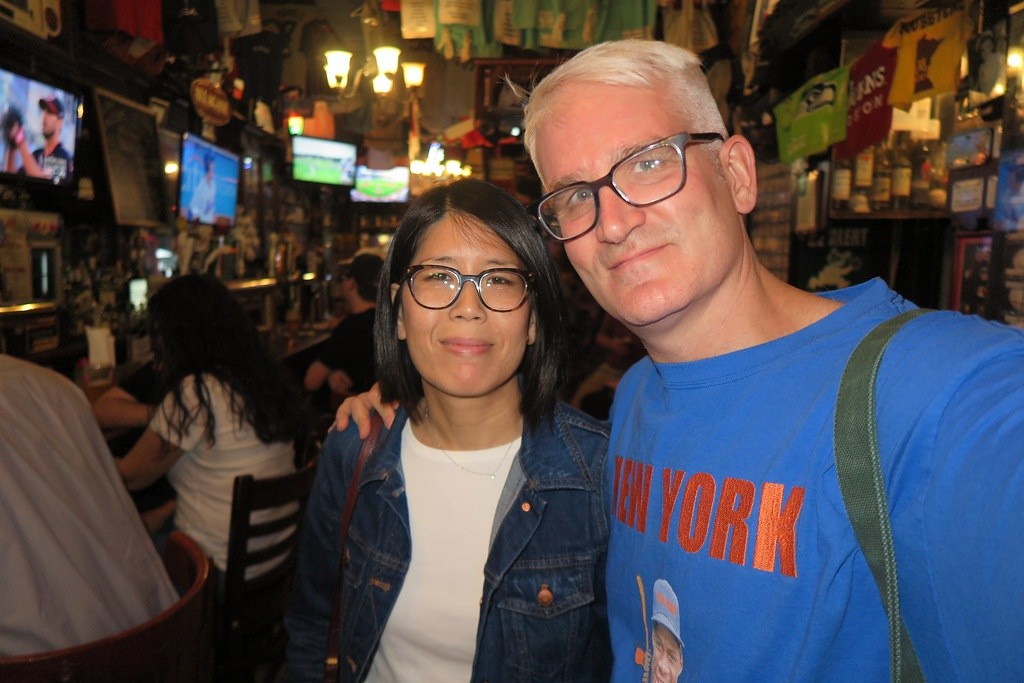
[39, 96, 65, 119]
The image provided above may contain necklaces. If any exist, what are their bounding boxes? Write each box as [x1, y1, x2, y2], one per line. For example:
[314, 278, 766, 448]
[424, 404, 523, 480]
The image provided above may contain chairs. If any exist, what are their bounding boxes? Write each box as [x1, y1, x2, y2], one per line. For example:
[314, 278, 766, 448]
[0, 429, 321, 683]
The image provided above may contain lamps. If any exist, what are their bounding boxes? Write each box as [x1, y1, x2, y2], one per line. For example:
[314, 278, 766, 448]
[322, 1, 427, 102]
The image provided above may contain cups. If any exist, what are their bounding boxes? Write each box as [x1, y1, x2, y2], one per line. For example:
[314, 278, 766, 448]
[82, 361, 115, 399]
[283, 314, 301, 340]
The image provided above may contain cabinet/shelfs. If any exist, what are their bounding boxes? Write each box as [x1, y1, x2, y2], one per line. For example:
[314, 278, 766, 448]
[474, 57, 563, 198]
[749, 0, 1024, 325]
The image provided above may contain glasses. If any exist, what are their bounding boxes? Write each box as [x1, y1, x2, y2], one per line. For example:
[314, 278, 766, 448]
[400, 264, 535, 313]
[526, 130, 725, 243]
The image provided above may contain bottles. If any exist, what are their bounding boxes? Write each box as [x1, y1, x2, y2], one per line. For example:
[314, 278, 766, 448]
[831, 130, 931, 211]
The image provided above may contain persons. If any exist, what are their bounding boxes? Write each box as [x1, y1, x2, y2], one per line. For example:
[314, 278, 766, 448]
[285, 255, 386, 432]
[6, 98, 73, 180]
[189, 154, 215, 223]
[288, 179, 613, 683]
[567, 311, 647, 409]
[94, 271, 298, 581]
[328, 39, 1024, 683]
[0, 353, 181, 656]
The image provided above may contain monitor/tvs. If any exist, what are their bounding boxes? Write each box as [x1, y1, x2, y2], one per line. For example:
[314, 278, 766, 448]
[0, 56, 90, 213]
[177, 129, 243, 229]
[291, 135, 358, 188]
[350, 165, 410, 203]
